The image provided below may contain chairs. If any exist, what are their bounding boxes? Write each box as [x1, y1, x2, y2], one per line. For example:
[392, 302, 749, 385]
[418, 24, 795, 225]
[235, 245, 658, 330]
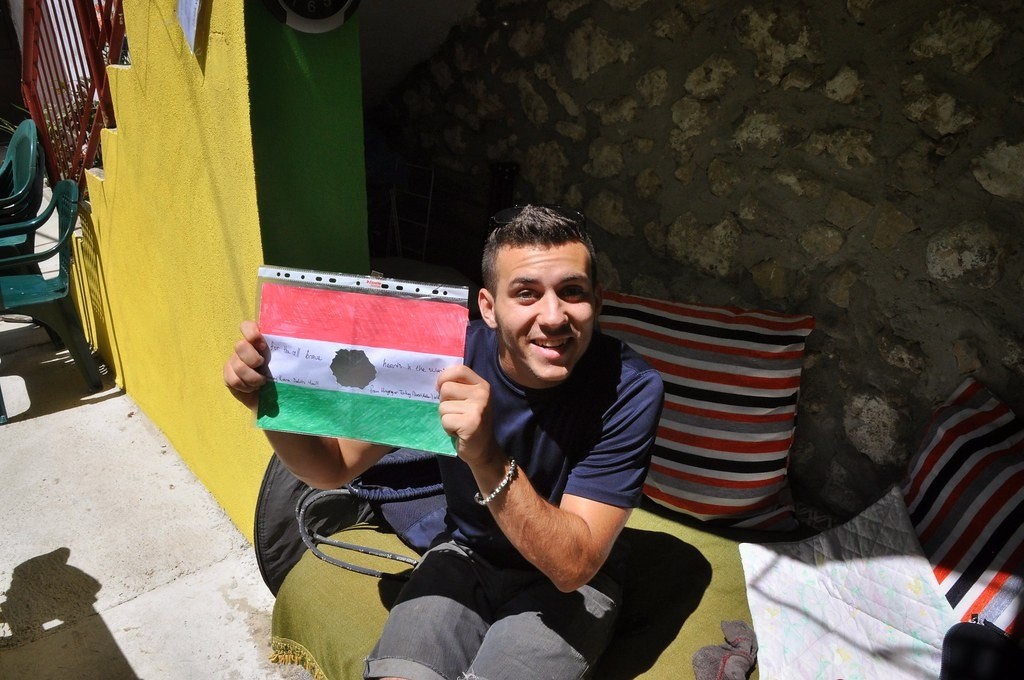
[0, 118, 46, 326]
[0, 179, 104, 426]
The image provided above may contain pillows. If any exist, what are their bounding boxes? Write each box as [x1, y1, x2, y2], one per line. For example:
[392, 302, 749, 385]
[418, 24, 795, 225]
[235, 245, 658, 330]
[902, 375, 1024, 643]
[597, 292, 815, 533]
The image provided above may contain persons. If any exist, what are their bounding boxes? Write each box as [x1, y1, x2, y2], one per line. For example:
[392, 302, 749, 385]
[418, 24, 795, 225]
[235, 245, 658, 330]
[224, 205, 664, 680]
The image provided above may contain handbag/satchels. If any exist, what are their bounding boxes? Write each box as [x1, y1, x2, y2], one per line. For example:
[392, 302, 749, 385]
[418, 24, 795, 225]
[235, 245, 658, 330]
[295, 448, 458, 585]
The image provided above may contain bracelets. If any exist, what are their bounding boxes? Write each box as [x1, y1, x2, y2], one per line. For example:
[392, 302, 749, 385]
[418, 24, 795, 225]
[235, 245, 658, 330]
[473, 453, 518, 506]
